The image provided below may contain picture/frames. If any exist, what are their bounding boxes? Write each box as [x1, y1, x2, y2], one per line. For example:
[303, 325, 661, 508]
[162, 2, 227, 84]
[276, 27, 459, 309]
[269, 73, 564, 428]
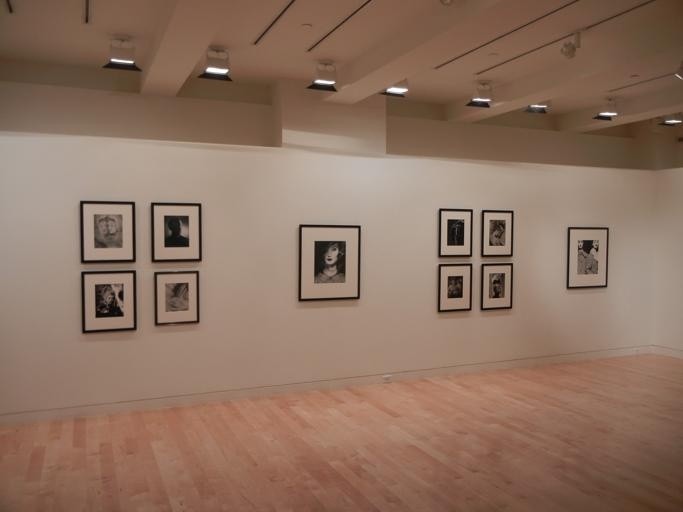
[480, 262, 513, 310]
[80, 270, 135, 333]
[438, 207, 473, 257]
[438, 263, 472, 313]
[566, 226, 609, 289]
[298, 223, 360, 302]
[154, 271, 199, 326]
[149, 201, 202, 263]
[80, 199, 135, 263]
[481, 209, 514, 258]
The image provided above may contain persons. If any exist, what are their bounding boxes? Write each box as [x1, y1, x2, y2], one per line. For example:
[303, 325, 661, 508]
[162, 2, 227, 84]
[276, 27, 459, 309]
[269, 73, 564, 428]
[97, 286, 123, 317]
[578, 240, 599, 274]
[314, 243, 346, 283]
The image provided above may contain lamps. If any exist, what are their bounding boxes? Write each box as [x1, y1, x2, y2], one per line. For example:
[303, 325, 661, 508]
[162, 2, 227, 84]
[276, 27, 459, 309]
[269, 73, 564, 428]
[592, 97, 619, 122]
[465, 79, 492, 109]
[307, 61, 337, 94]
[193, 51, 232, 83]
[524, 104, 548, 116]
[102, 37, 144, 76]
[659, 115, 682, 126]
[674, 70, 683, 80]
[379, 80, 408, 98]
